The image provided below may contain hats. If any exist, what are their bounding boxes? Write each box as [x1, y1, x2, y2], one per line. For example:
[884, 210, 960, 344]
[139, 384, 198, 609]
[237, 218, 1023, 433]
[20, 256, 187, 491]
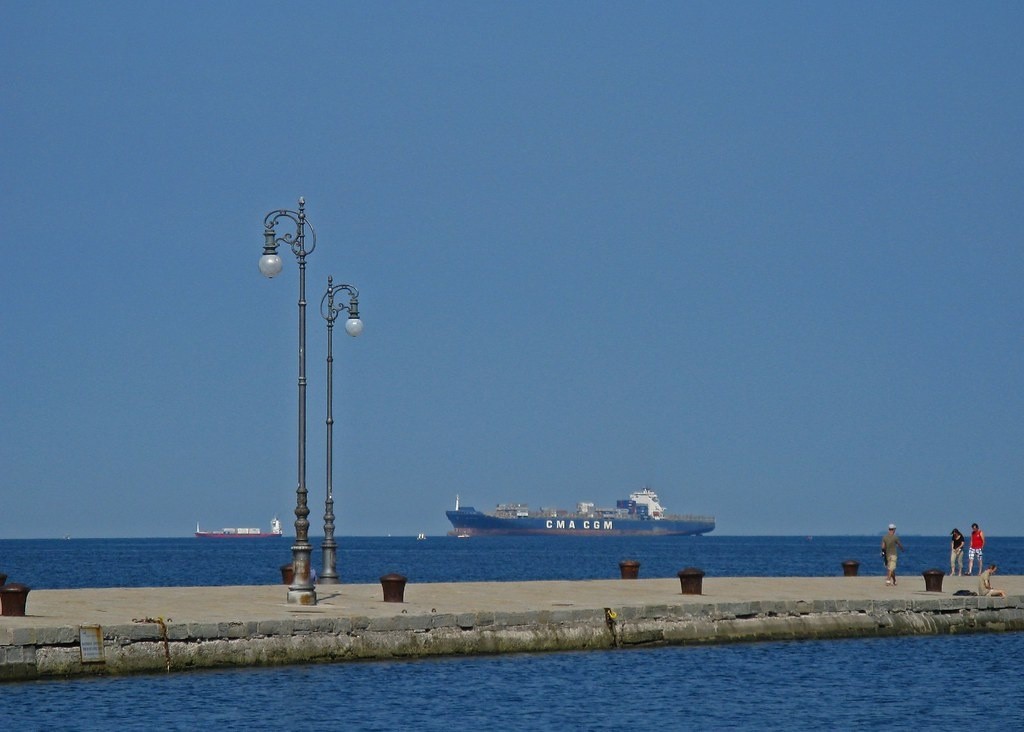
[888, 524, 896, 529]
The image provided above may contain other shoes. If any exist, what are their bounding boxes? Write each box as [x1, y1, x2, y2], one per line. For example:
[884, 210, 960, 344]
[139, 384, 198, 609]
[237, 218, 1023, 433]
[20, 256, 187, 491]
[885, 580, 897, 586]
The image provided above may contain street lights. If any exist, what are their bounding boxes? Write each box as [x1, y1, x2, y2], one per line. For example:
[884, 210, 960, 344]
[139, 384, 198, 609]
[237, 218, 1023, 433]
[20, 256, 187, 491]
[260, 193, 316, 605]
[315, 274, 363, 586]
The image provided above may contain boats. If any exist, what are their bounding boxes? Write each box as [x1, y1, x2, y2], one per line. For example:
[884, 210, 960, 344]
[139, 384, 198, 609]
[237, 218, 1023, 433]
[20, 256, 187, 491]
[195, 514, 282, 539]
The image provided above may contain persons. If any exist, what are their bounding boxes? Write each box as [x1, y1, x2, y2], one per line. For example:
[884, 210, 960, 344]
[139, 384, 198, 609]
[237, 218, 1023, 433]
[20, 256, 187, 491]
[965, 523, 985, 576]
[950, 528, 965, 575]
[882, 524, 904, 585]
[978, 565, 1013, 607]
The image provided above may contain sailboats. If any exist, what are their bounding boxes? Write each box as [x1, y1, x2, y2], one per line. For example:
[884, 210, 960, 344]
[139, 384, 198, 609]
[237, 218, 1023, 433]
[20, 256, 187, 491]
[417, 534, 427, 540]
[458, 531, 470, 538]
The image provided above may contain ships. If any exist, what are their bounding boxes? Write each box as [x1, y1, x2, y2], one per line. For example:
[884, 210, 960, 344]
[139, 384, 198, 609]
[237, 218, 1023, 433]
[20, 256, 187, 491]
[445, 487, 716, 538]
